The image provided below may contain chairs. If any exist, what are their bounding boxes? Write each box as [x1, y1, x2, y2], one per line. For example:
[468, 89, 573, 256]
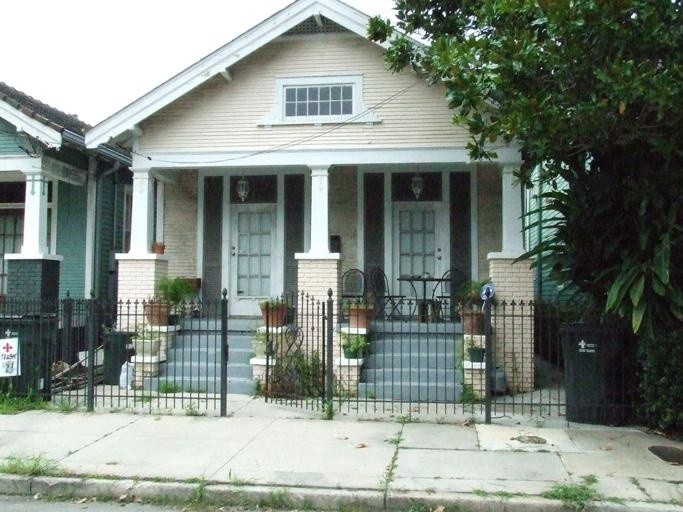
[341, 268, 467, 325]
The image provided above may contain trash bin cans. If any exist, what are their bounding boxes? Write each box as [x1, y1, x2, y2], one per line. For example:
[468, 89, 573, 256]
[101, 332, 138, 385]
[0, 313, 58, 402]
[558, 314, 632, 427]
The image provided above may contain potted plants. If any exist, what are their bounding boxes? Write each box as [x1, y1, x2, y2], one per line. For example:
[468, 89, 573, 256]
[130, 295, 171, 357]
[459, 310, 486, 361]
[340, 298, 373, 357]
[251, 298, 286, 359]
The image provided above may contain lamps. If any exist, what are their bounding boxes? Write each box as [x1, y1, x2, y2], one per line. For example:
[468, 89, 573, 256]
[408, 163, 424, 201]
[234, 168, 248, 202]
